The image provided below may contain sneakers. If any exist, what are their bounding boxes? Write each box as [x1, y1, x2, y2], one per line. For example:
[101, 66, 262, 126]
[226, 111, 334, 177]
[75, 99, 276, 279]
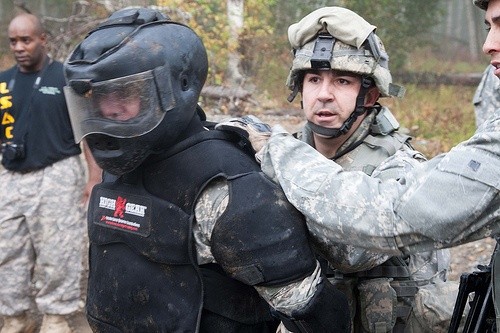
[0, 311, 36, 333]
[39, 314, 71, 333]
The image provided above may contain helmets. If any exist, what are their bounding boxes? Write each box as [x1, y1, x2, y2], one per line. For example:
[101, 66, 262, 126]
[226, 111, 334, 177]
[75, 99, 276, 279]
[63, 8, 208, 175]
[287, 32, 407, 102]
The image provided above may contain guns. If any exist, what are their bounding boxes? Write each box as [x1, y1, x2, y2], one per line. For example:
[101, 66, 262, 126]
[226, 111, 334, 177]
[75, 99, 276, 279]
[448, 240, 497, 333]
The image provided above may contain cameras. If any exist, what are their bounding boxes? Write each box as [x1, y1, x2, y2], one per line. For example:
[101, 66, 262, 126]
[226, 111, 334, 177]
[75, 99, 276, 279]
[1, 142, 25, 160]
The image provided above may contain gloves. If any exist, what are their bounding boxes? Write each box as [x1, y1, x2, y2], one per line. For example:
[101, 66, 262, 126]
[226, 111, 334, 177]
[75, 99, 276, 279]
[215, 115, 273, 165]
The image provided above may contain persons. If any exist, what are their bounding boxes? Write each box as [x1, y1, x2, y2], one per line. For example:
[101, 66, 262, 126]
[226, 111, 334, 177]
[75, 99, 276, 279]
[62, 8, 351, 333]
[214, 0, 500, 333]
[0, 13, 103, 333]
[276, 6, 451, 333]
